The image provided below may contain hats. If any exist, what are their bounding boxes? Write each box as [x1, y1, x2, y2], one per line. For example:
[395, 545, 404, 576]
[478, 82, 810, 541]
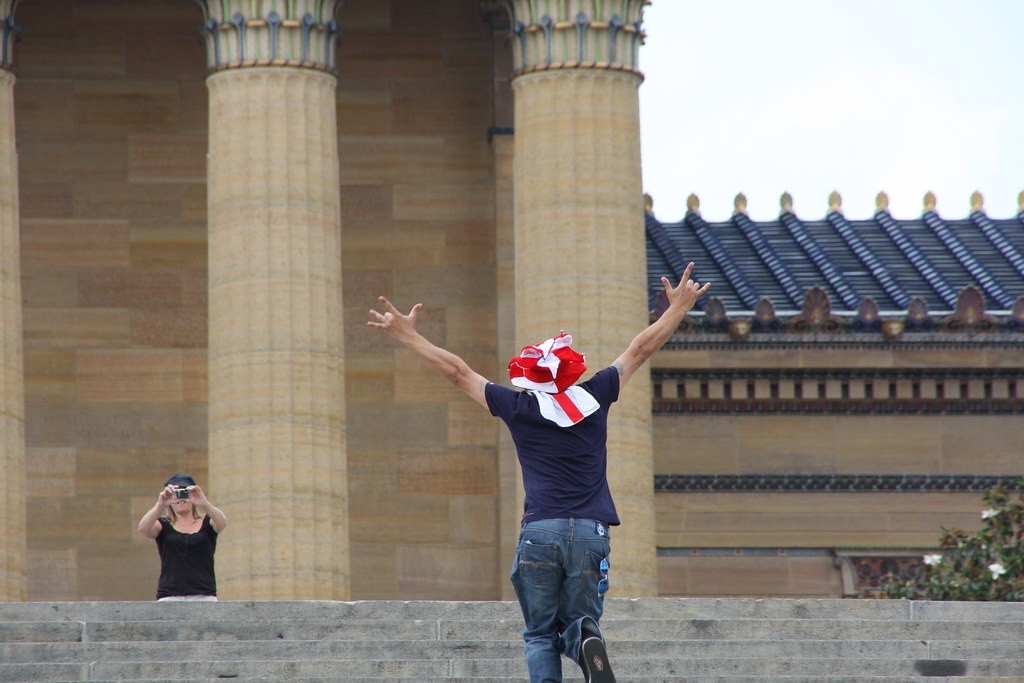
[164, 472, 198, 488]
[508, 330, 586, 393]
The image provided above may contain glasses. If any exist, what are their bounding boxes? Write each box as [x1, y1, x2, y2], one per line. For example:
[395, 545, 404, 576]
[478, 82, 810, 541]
[597, 559, 610, 598]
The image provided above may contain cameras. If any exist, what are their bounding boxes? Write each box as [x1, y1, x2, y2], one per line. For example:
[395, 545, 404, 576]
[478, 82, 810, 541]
[173, 488, 189, 500]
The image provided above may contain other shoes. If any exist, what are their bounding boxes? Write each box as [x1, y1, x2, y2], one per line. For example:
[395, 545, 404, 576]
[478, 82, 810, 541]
[579, 635, 616, 683]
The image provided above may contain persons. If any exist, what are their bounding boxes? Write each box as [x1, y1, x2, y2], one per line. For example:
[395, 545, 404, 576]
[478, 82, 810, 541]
[366, 262, 710, 683]
[138, 474, 227, 602]
